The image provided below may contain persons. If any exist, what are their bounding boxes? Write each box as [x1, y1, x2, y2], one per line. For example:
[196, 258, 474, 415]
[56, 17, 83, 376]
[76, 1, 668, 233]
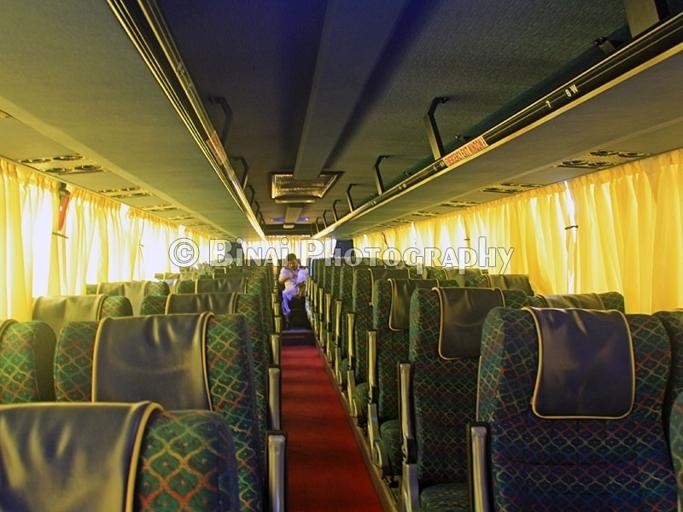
[278, 253, 309, 331]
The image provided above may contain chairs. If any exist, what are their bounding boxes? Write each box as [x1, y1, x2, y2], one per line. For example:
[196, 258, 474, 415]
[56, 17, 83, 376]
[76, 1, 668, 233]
[0, 257, 683, 512]
